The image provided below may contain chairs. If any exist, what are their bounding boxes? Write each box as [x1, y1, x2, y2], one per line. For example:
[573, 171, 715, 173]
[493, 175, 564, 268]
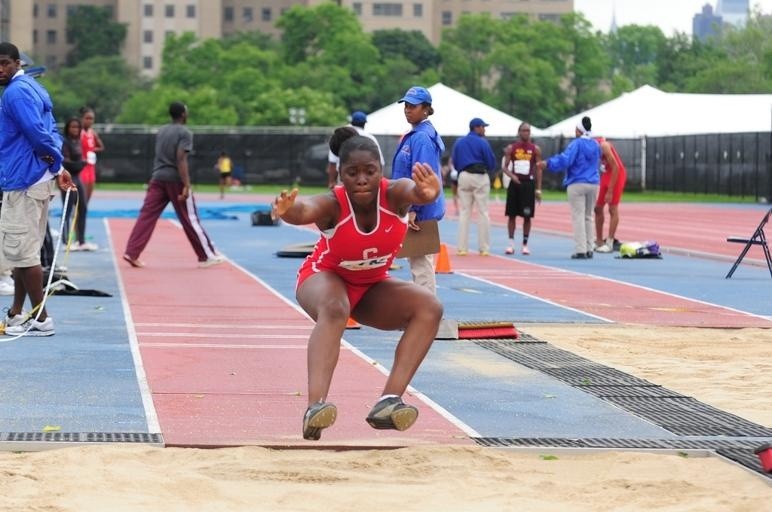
[726, 209, 772, 278]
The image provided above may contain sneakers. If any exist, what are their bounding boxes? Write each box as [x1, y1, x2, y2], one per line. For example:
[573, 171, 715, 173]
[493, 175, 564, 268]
[4, 241, 142, 336]
[303, 401, 337, 441]
[458, 243, 614, 259]
[197, 255, 225, 268]
[366, 396, 418, 431]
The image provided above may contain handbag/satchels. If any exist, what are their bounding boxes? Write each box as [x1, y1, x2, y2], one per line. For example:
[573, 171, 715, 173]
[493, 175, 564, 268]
[465, 164, 486, 174]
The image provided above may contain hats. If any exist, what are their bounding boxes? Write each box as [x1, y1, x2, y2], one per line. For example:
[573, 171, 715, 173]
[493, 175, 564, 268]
[351, 112, 367, 122]
[398, 87, 432, 105]
[470, 118, 489, 127]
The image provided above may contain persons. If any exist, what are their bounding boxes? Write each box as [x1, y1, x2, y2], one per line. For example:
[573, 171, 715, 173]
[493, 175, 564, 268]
[389, 86, 447, 326]
[500, 118, 544, 258]
[271, 134, 443, 442]
[589, 135, 627, 253]
[535, 121, 603, 261]
[76, 106, 107, 207]
[0, 42, 78, 337]
[121, 101, 229, 270]
[214, 152, 236, 200]
[327, 111, 385, 187]
[59, 106, 100, 253]
[450, 118, 497, 256]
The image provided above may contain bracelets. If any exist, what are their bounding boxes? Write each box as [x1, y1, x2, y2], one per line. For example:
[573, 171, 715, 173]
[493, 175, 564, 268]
[51, 165, 64, 177]
